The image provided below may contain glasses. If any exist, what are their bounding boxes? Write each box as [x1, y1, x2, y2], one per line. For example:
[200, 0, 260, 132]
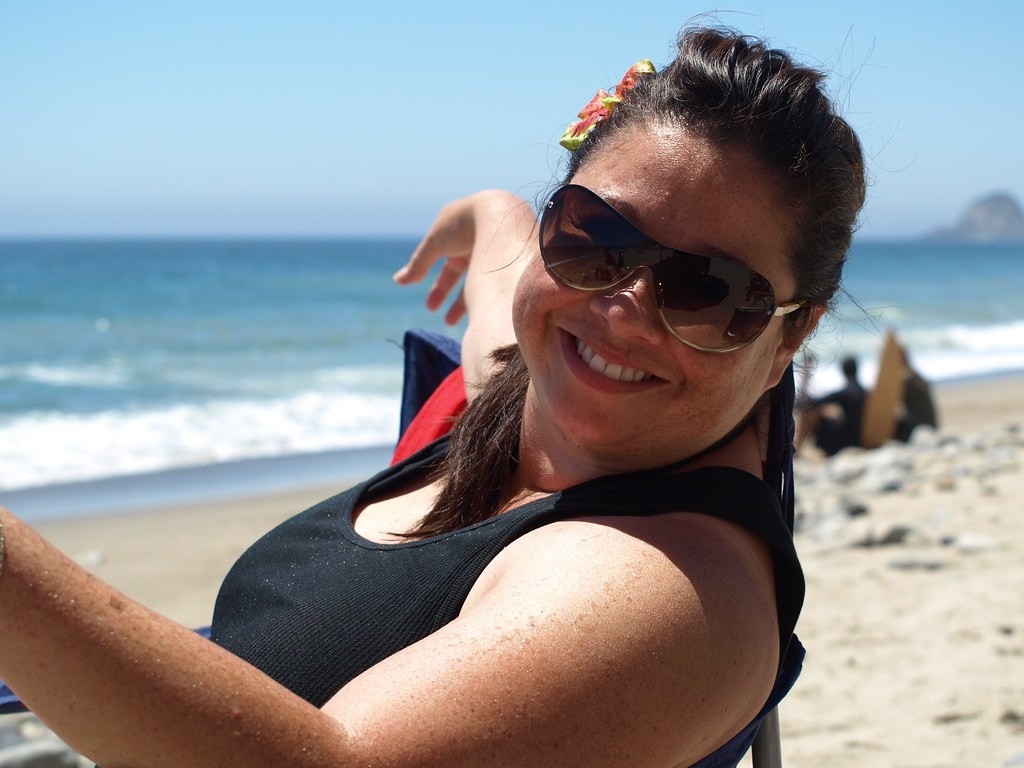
[538, 186, 807, 356]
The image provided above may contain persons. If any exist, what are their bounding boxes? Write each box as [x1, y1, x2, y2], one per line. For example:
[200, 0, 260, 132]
[0, 19, 865, 768]
[789, 355, 870, 455]
